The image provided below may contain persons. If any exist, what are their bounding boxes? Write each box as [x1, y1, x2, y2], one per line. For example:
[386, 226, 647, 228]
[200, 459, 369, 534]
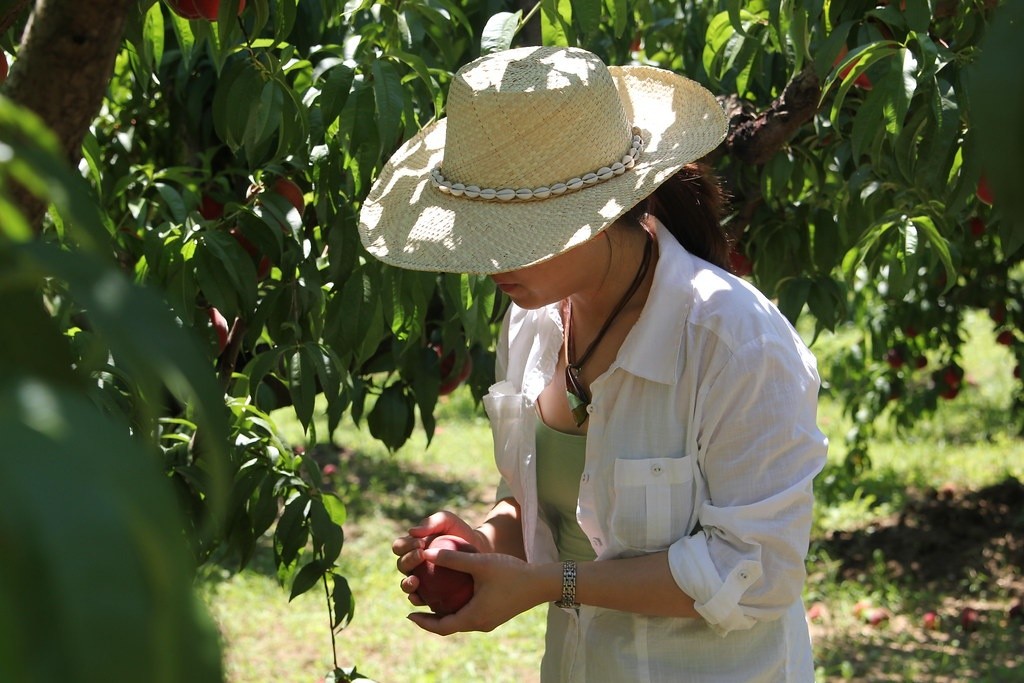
[357, 44, 825, 681]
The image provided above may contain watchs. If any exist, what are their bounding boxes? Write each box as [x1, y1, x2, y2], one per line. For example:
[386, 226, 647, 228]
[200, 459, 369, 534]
[554, 560, 582, 609]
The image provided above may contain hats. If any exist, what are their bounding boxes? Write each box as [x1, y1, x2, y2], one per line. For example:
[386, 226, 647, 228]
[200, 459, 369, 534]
[358, 46, 730, 276]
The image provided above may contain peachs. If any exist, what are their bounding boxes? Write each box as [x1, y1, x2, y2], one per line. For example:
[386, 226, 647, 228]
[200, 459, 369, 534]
[0, 0, 1023, 403]
[413, 536, 479, 614]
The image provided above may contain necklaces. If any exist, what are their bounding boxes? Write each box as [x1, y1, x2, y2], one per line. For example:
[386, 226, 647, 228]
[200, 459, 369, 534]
[562, 229, 653, 428]
